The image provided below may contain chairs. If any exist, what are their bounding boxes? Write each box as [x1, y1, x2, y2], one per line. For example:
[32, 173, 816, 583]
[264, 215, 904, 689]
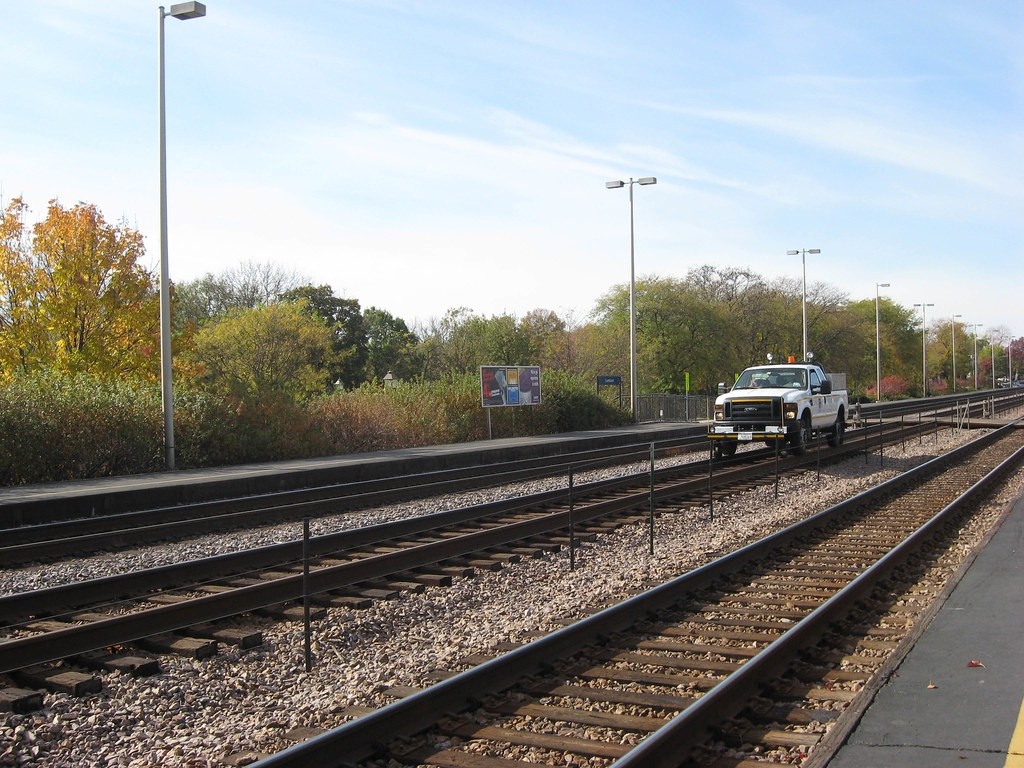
[751, 379, 772, 388]
[783, 373, 805, 387]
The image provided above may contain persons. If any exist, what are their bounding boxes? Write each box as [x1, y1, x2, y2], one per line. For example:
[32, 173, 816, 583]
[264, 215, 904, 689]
[750, 374, 767, 388]
[784, 371, 805, 387]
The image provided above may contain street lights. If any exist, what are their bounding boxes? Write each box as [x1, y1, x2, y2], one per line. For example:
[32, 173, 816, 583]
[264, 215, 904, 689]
[969, 323, 984, 390]
[159, 0, 205, 474]
[991, 329, 1000, 390]
[875, 282, 891, 402]
[1006, 335, 1015, 388]
[786, 248, 821, 362]
[604, 178, 658, 421]
[951, 314, 962, 394]
[913, 303, 935, 398]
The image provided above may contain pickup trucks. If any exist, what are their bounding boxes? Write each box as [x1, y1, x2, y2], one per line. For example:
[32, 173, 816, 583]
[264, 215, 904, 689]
[706, 352, 849, 462]
